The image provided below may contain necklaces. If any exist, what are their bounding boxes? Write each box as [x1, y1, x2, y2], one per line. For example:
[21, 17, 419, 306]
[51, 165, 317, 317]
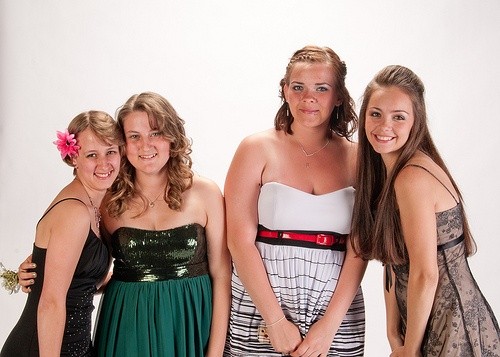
[135, 177, 167, 208]
[292, 131, 332, 168]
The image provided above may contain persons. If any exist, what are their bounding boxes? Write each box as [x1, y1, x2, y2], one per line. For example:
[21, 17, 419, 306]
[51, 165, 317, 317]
[95, 92, 233, 357]
[0, 109, 122, 357]
[221, 46, 364, 357]
[351, 65, 500, 357]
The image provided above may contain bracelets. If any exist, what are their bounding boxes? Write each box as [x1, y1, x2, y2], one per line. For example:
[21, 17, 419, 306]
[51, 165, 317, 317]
[265, 315, 286, 325]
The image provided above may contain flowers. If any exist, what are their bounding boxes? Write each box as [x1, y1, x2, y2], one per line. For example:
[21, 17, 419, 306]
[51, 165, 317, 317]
[53, 129, 80, 160]
